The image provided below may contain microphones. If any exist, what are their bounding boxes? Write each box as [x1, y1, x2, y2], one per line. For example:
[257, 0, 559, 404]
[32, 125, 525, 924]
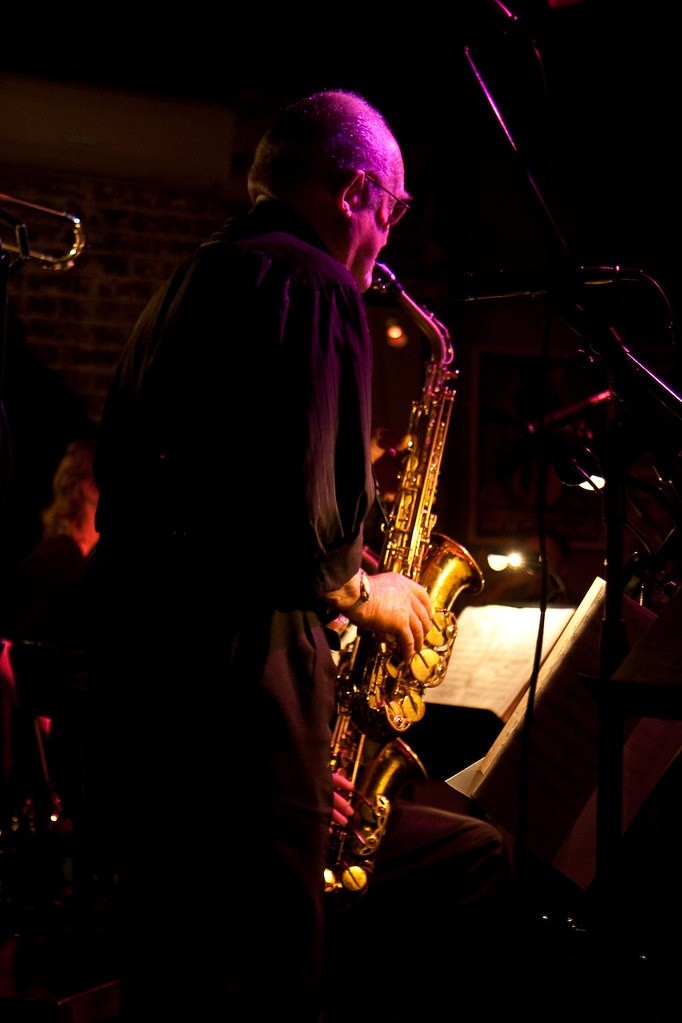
[580, 266, 643, 277]
[527, 390, 614, 436]
[490, 0, 541, 58]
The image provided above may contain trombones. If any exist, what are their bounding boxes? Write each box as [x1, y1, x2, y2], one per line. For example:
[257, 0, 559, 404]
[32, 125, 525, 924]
[0, 190, 86, 274]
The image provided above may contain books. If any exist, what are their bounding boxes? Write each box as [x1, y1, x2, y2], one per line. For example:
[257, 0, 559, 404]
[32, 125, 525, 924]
[424, 578, 665, 862]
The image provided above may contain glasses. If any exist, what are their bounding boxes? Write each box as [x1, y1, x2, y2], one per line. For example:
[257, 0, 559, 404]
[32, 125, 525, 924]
[340, 172, 410, 227]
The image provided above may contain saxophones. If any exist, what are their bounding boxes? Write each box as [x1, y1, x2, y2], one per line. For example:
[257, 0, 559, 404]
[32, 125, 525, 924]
[315, 258, 487, 896]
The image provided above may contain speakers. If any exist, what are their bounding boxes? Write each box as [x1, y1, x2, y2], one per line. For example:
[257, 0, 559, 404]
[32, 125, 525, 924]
[552, 716, 682, 891]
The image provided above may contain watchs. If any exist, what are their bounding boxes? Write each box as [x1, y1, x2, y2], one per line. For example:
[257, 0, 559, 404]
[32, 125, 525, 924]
[344, 569, 371, 613]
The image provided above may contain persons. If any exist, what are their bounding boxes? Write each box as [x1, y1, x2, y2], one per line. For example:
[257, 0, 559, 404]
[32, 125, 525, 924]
[22, 435, 100, 565]
[90, 87, 456, 1023]
